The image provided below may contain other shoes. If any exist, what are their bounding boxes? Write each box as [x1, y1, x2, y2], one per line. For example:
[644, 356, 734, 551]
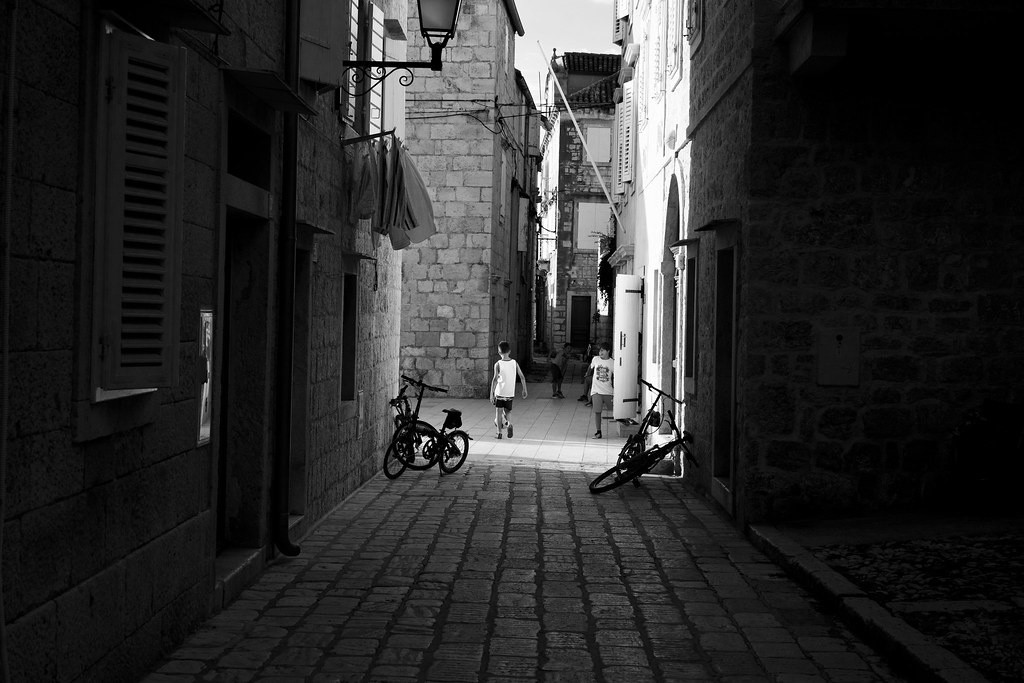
[493, 418, 503, 428]
[556, 390, 566, 398]
[552, 394, 559, 398]
[507, 424, 513, 438]
[503, 414, 508, 425]
[585, 398, 592, 406]
[592, 430, 602, 439]
[495, 433, 502, 439]
[578, 394, 588, 401]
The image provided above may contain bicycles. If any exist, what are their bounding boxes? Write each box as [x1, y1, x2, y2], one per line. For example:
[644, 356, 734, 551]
[615, 377, 683, 477]
[389, 384, 443, 471]
[383, 374, 474, 480]
[589, 410, 702, 496]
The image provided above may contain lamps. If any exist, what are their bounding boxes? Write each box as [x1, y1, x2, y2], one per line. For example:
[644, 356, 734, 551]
[341, 0, 462, 96]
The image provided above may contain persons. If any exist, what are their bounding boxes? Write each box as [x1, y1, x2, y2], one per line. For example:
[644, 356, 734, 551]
[577, 343, 614, 439]
[490, 341, 527, 438]
[547, 342, 573, 399]
